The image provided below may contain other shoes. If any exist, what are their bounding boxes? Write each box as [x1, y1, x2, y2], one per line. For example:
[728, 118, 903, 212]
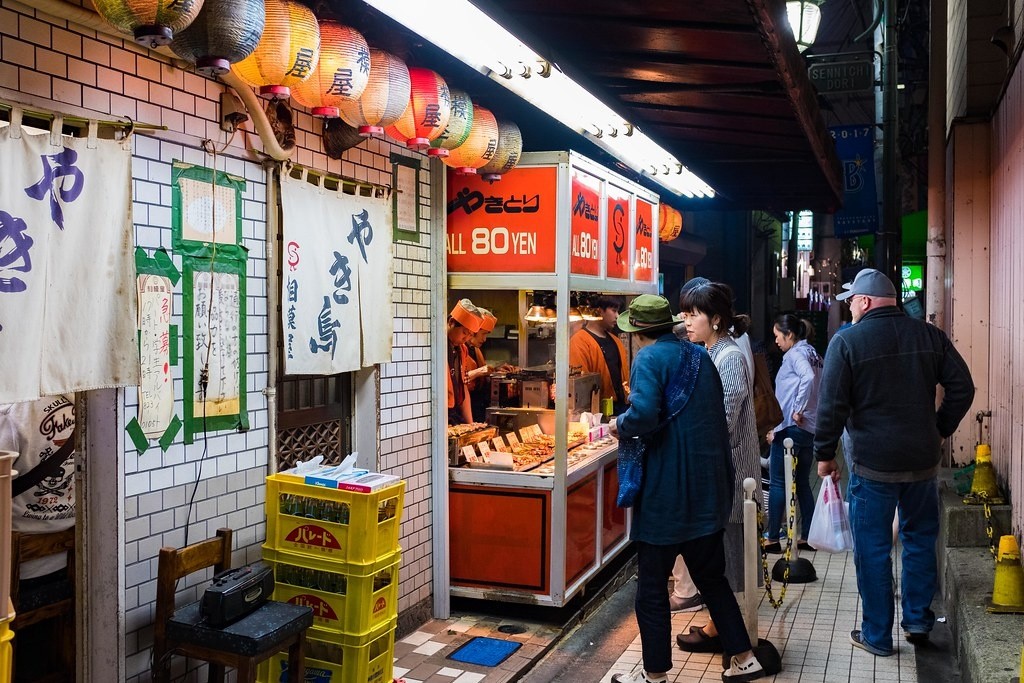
[798, 542, 817, 551]
[761, 542, 782, 554]
[669, 593, 703, 613]
[849, 630, 869, 652]
[905, 629, 930, 638]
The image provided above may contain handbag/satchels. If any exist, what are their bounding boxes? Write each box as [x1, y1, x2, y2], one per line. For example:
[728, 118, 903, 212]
[807, 475, 854, 554]
[753, 354, 784, 436]
[617, 438, 647, 507]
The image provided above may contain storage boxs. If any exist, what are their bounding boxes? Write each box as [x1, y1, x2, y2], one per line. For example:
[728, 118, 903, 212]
[252, 461, 406, 683]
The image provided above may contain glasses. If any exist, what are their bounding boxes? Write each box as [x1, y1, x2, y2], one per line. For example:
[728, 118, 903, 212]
[844, 296, 863, 305]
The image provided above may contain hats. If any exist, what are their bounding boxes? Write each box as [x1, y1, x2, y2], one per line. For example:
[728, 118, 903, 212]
[842, 268, 879, 289]
[617, 294, 684, 333]
[478, 307, 498, 333]
[836, 271, 897, 301]
[450, 298, 485, 333]
[682, 277, 711, 291]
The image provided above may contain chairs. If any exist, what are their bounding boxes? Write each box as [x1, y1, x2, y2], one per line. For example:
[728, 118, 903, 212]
[151, 528, 314, 682]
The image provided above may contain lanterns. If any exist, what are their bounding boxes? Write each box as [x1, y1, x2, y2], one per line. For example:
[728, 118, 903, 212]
[659, 201, 682, 244]
[91, 0, 523, 181]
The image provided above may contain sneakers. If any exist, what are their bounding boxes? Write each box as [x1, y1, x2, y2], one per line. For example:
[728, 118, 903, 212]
[611, 669, 669, 683]
[722, 655, 765, 681]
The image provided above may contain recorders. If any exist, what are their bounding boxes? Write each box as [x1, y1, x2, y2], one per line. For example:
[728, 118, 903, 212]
[197, 561, 275, 629]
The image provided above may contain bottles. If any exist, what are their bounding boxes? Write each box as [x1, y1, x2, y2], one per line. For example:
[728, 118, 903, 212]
[275, 563, 391, 595]
[279, 492, 397, 526]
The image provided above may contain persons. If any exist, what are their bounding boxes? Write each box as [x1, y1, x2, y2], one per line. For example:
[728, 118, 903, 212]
[569, 294, 631, 416]
[668, 276, 824, 654]
[447, 298, 515, 426]
[836, 315, 899, 596]
[608, 294, 765, 683]
[814, 268, 975, 656]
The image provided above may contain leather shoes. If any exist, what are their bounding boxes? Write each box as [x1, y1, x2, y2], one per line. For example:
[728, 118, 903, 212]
[676, 628, 723, 652]
[690, 625, 707, 632]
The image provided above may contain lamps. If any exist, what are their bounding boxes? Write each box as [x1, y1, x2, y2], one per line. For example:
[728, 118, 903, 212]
[524, 290, 604, 322]
[786, 0, 822, 55]
